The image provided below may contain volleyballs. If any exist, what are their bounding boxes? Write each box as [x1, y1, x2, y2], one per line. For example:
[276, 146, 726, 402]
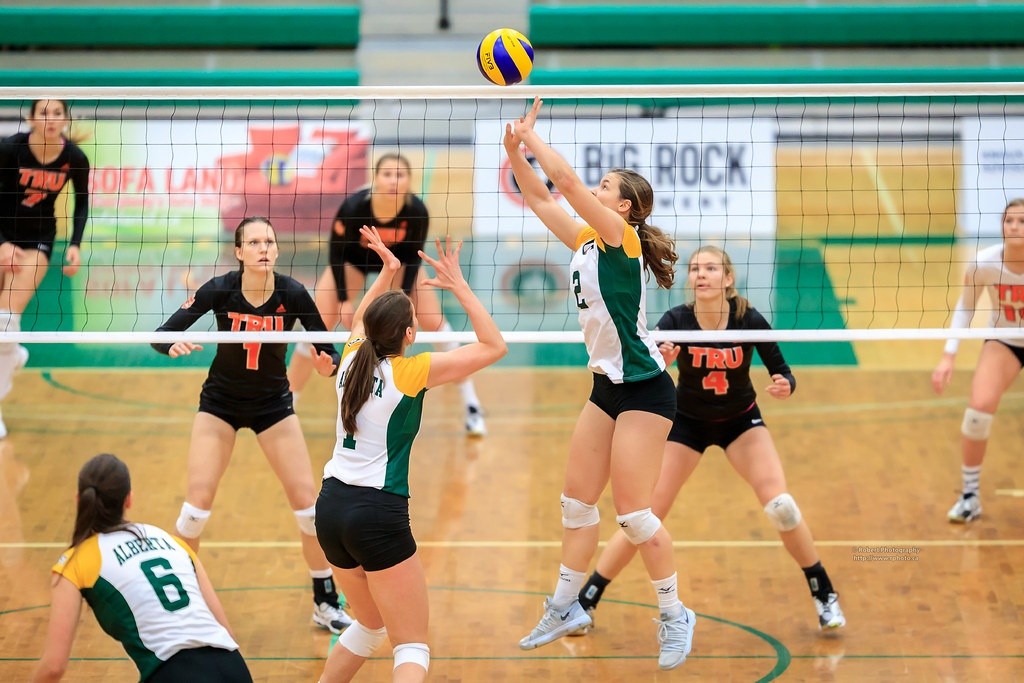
[477, 28, 537, 85]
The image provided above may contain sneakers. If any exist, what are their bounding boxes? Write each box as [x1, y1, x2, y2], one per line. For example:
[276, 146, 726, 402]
[568, 607, 594, 635]
[519, 595, 591, 650]
[313, 598, 353, 635]
[651, 606, 696, 671]
[946, 495, 982, 522]
[814, 593, 845, 631]
[466, 403, 485, 436]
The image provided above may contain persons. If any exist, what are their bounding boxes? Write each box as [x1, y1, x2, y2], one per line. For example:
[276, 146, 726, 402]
[1, 99, 90, 439]
[932, 197, 1024, 523]
[151, 216, 354, 635]
[556, 246, 847, 635]
[285, 155, 489, 440]
[33, 453, 254, 682]
[312, 224, 510, 683]
[505, 95, 697, 672]
